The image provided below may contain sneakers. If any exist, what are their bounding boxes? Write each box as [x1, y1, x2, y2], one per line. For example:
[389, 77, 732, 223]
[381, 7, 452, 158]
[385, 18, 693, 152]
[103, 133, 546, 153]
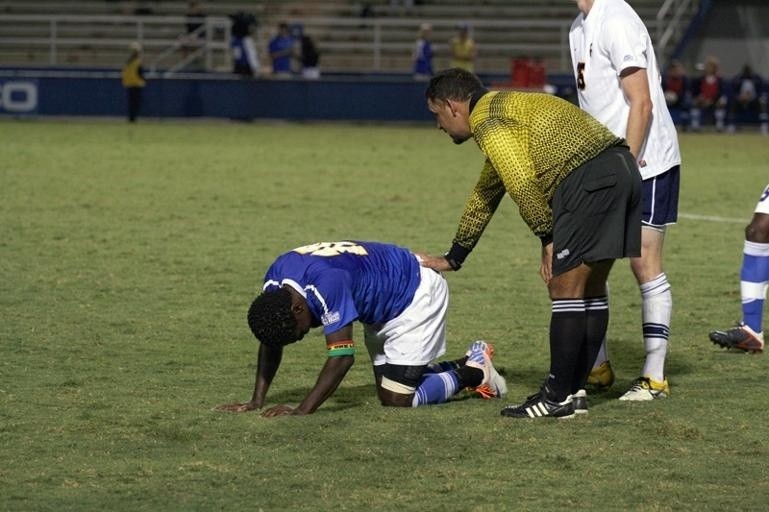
[466, 340, 495, 392]
[465, 348, 508, 400]
[618, 375, 670, 402]
[527, 389, 588, 415]
[500, 391, 576, 420]
[584, 361, 615, 390]
[708, 322, 764, 353]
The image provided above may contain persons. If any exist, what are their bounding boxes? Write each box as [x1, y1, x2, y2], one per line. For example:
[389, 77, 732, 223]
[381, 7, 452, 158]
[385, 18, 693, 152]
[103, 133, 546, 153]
[413, 67, 642, 421]
[710, 184, 769, 355]
[450, 23, 477, 74]
[122, 42, 153, 122]
[411, 21, 436, 82]
[663, 58, 769, 134]
[229, 9, 319, 79]
[211, 240, 508, 420]
[183, 2, 207, 64]
[568, 0, 682, 402]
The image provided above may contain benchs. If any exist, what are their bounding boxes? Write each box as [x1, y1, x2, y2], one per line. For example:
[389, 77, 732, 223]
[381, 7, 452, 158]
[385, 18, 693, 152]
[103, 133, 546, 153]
[0, 0, 692, 77]
